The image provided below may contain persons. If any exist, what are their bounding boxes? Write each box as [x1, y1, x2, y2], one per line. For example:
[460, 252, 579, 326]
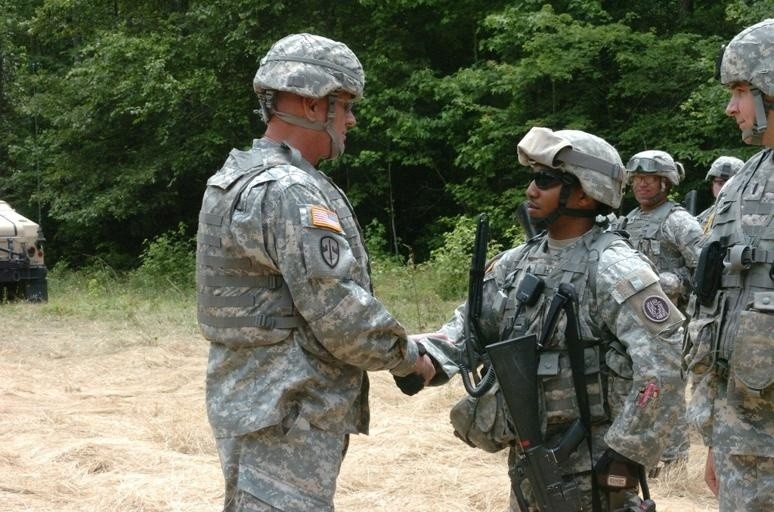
[700, 18, 774, 510]
[688, 156, 747, 443]
[610, 149, 707, 480]
[193, 33, 450, 511]
[394, 126, 685, 510]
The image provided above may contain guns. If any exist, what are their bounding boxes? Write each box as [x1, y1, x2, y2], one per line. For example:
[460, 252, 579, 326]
[486, 334, 588, 510]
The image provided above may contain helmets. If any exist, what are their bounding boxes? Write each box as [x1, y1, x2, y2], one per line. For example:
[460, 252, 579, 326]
[704, 156, 744, 181]
[529, 132, 624, 210]
[720, 18, 773, 93]
[627, 151, 680, 185]
[253, 34, 365, 100]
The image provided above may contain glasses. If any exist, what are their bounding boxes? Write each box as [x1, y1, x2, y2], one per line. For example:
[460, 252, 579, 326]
[527, 170, 564, 190]
[627, 158, 674, 172]
[631, 175, 659, 183]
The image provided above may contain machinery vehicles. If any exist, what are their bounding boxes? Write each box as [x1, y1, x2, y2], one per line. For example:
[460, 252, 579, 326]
[0, 197, 52, 305]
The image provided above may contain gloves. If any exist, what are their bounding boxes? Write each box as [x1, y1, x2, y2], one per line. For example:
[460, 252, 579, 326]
[592, 449, 640, 493]
[393, 342, 426, 397]
